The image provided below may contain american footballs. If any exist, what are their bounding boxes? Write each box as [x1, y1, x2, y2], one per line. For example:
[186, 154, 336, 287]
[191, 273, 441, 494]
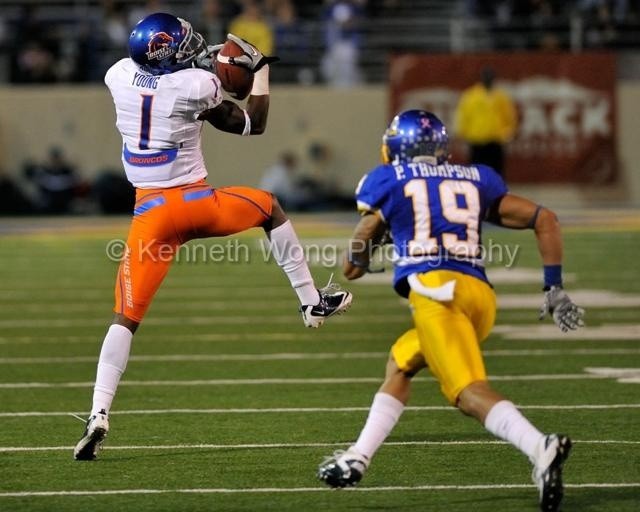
[217, 39, 253, 101]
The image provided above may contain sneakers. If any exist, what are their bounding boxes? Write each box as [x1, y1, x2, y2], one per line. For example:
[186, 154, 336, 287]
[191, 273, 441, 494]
[317, 446, 368, 488]
[531, 434, 572, 512]
[74, 409, 110, 459]
[298, 283, 352, 329]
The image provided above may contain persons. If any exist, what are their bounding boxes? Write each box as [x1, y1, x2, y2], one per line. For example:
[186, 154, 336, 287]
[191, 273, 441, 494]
[254, 147, 319, 211]
[73, 13, 357, 465]
[68, 182, 105, 216]
[451, 65, 520, 181]
[297, 139, 362, 209]
[36, 144, 80, 214]
[313, 102, 592, 508]
[11, 1, 640, 81]
[17, 161, 50, 216]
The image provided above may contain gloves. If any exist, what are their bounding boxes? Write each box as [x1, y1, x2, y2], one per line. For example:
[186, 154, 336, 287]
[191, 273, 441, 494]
[539, 285, 585, 332]
[191, 44, 224, 69]
[227, 33, 279, 72]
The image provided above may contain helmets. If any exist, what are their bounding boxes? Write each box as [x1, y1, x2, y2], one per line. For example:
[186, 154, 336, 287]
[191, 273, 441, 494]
[381, 108, 449, 167]
[127, 12, 208, 69]
